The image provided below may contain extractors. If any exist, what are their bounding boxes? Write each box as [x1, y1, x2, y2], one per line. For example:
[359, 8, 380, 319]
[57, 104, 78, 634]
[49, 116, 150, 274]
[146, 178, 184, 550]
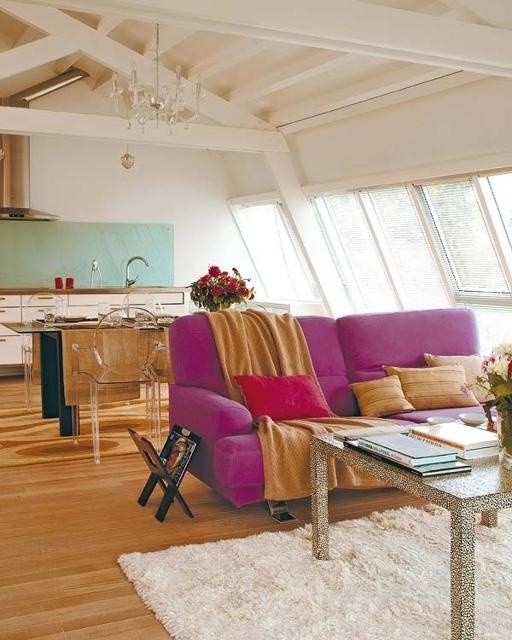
[0, 70, 88, 221]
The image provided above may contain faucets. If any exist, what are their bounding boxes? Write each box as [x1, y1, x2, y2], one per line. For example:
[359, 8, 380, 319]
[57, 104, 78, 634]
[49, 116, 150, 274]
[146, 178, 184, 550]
[125, 256, 149, 288]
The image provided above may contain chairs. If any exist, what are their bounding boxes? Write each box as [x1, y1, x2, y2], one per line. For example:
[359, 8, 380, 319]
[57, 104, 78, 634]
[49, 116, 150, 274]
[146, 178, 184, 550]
[21, 288, 71, 411]
[121, 289, 161, 318]
[77, 306, 161, 464]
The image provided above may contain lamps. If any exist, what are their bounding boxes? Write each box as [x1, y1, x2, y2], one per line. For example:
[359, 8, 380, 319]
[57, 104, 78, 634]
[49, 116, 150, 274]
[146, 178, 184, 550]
[120, 143, 135, 169]
[111, 24, 204, 134]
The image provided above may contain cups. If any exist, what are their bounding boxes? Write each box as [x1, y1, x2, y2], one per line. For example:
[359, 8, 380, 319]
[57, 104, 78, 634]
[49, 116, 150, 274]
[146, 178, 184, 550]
[134, 310, 148, 322]
[98, 302, 110, 322]
[43, 307, 56, 326]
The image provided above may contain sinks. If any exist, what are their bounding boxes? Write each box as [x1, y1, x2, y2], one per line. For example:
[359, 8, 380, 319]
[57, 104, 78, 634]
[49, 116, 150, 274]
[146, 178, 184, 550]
[132, 286, 168, 288]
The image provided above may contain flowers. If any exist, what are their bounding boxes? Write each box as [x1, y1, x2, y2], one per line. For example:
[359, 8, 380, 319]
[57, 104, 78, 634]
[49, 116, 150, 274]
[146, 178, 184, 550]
[185, 266, 256, 311]
[465, 344, 512, 458]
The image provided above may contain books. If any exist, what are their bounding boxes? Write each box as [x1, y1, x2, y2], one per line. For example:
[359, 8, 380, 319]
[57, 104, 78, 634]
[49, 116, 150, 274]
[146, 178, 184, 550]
[343, 433, 472, 477]
[409, 423, 500, 460]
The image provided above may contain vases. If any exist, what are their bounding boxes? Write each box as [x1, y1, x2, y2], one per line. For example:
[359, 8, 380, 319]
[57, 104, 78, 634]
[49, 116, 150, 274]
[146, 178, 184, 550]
[498, 411, 512, 468]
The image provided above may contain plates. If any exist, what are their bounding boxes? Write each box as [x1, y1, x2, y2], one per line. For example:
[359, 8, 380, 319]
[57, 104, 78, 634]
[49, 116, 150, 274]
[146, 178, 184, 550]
[459, 413, 485, 425]
[427, 416, 454, 425]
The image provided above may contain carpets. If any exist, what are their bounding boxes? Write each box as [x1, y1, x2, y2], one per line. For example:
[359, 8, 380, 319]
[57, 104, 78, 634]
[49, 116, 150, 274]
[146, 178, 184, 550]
[118, 504, 512, 639]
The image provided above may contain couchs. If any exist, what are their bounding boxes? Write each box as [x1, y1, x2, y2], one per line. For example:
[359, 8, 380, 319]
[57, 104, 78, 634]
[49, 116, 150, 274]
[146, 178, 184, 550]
[169, 308, 498, 508]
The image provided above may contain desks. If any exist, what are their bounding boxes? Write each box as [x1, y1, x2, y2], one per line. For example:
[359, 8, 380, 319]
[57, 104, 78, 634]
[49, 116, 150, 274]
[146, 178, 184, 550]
[0, 316, 183, 437]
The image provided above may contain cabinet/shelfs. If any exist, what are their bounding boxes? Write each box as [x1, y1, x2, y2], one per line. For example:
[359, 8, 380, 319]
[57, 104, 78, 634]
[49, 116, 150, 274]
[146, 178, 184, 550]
[1, 294, 68, 363]
[128, 293, 184, 317]
[69, 294, 128, 319]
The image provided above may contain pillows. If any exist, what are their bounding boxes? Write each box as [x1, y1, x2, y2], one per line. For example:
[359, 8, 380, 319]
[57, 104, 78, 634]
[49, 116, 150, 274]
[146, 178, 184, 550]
[234, 374, 333, 426]
[382, 363, 480, 409]
[349, 374, 415, 418]
[423, 353, 497, 402]
